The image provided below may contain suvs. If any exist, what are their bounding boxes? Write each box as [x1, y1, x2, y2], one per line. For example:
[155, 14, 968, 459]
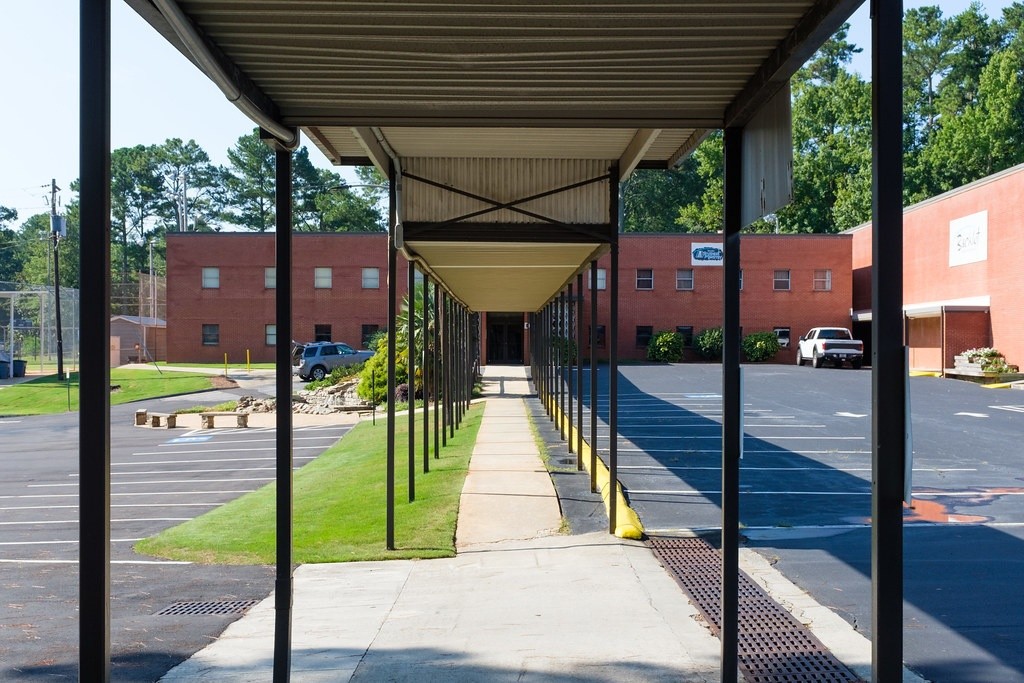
[292, 340, 376, 382]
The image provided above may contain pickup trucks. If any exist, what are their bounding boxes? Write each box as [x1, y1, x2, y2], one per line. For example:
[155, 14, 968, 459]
[775, 329, 788, 347]
[796, 327, 864, 370]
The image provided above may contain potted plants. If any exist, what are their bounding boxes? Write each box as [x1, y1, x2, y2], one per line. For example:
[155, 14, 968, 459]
[954, 347, 1018, 374]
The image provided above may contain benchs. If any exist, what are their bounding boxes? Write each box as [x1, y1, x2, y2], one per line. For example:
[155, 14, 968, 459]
[200, 413, 249, 429]
[151, 413, 177, 428]
[135, 409, 147, 425]
